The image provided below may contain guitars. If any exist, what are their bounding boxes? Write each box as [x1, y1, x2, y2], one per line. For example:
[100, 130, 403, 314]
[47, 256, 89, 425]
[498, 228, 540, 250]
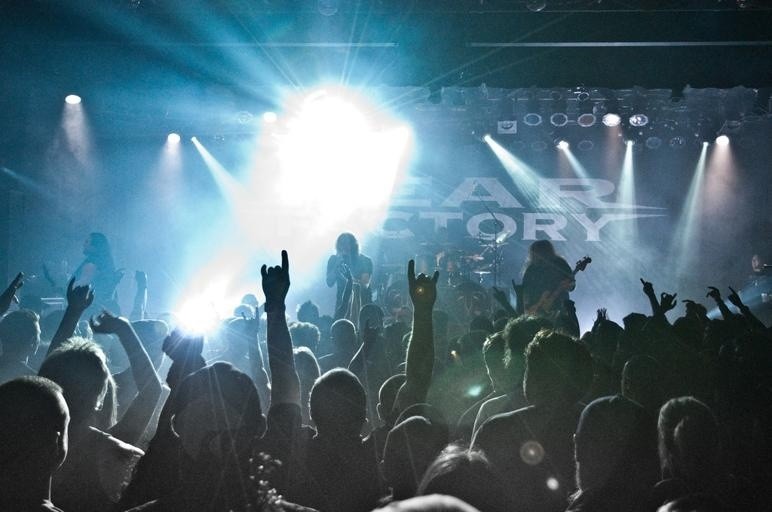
[526, 256, 591, 314]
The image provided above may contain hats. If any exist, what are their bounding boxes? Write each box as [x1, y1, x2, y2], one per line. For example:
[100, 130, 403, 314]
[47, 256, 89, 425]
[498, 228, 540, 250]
[174, 361, 261, 431]
[576, 392, 653, 452]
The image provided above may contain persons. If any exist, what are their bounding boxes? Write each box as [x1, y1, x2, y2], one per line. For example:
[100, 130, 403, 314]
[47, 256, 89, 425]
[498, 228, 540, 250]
[0, 233, 772, 512]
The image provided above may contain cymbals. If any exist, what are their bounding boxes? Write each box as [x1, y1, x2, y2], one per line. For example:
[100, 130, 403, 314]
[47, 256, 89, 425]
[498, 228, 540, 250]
[465, 236, 495, 241]
